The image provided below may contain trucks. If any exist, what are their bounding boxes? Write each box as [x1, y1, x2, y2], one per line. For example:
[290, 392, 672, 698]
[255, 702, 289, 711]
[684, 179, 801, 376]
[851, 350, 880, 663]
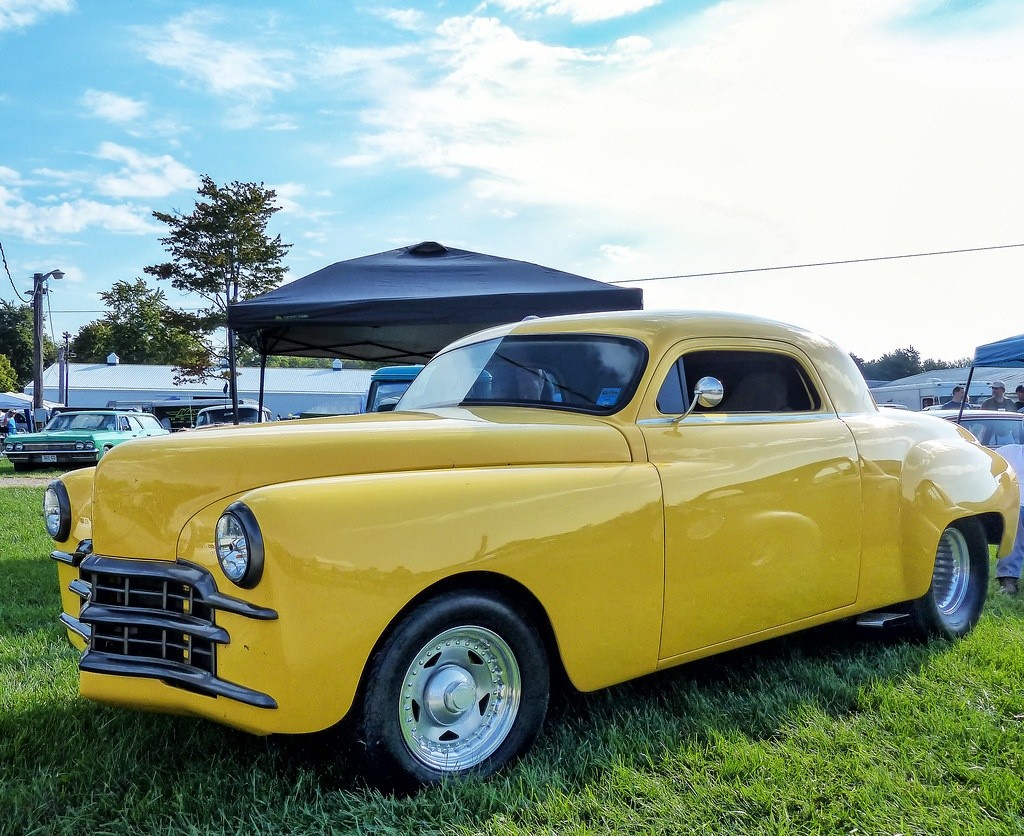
[868, 377, 995, 414]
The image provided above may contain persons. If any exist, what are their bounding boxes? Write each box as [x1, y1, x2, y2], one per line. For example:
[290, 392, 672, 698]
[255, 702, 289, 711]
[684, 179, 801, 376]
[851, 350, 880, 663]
[3, 409, 17, 436]
[160, 412, 172, 432]
[995, 506, 1024, 598]
[121, 410, 133, 427]
[941, 386, 974, 431]
[492, 314, 555, 402]
[980, 379, 1024, 444]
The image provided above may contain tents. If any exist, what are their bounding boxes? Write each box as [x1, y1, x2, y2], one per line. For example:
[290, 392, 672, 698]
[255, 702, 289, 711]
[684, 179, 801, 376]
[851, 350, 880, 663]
[956, 333, 1024, 423]
[220, 243, 643, 429]
[0, 391, 65, 417]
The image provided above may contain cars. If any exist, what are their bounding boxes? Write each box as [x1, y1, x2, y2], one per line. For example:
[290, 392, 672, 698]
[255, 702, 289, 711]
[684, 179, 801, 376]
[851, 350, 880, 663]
[37, 306, 1022, 795]
[915, 408, 1024, 451]
[3, 409, 171, 471]
[194, 399, 272, 429]
[362, 363, 494, 414]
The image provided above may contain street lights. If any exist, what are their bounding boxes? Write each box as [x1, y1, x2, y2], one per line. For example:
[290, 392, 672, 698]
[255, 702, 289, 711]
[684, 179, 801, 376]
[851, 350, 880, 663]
[24, 267, 65, 430]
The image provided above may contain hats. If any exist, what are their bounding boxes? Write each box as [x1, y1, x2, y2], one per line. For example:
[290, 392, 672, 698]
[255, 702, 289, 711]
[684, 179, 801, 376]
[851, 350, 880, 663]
[989, 380, 1005, 388]
